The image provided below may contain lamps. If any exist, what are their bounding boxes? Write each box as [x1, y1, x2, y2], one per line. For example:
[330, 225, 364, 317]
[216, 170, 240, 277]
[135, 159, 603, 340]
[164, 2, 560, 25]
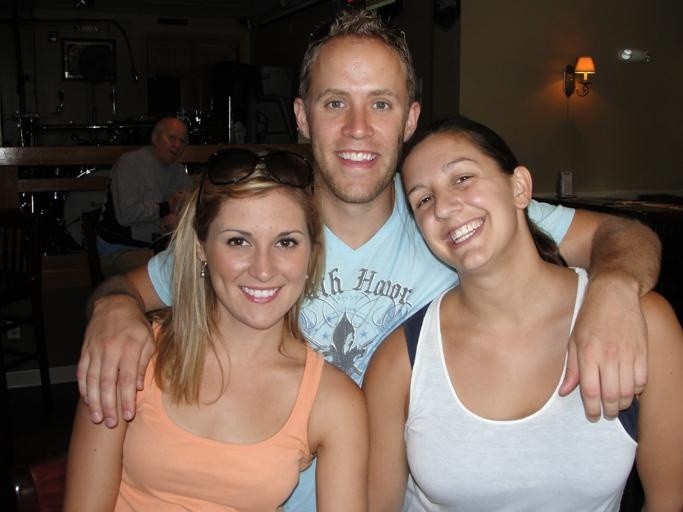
[563, 57, 596, 98]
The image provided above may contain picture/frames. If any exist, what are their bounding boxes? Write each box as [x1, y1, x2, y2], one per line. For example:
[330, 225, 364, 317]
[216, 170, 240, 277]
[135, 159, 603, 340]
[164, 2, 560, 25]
[60, 38, 117, 82]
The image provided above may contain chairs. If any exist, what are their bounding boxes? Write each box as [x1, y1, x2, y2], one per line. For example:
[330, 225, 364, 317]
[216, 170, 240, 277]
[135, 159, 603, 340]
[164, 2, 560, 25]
[1, 209, 53, 410]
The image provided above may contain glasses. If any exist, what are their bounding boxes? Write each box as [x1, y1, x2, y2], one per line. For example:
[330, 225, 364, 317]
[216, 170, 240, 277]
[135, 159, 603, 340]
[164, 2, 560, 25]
[194, 147, 316, 211]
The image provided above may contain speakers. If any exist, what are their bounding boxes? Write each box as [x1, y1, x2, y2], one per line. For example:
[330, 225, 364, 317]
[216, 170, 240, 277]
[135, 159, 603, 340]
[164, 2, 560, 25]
[209, 64, 249, 120]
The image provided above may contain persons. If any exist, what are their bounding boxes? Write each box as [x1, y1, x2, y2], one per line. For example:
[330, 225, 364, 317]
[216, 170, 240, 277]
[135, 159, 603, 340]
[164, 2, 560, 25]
[76, 9, 662, 512]
[95, 117, 199, 275]
[63, 146, 370, 512]
[362, 115, 683, 512]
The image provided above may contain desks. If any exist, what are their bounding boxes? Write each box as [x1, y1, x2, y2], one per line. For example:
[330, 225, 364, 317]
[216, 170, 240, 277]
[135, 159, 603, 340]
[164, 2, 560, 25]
[532, 194, 683, 223]
